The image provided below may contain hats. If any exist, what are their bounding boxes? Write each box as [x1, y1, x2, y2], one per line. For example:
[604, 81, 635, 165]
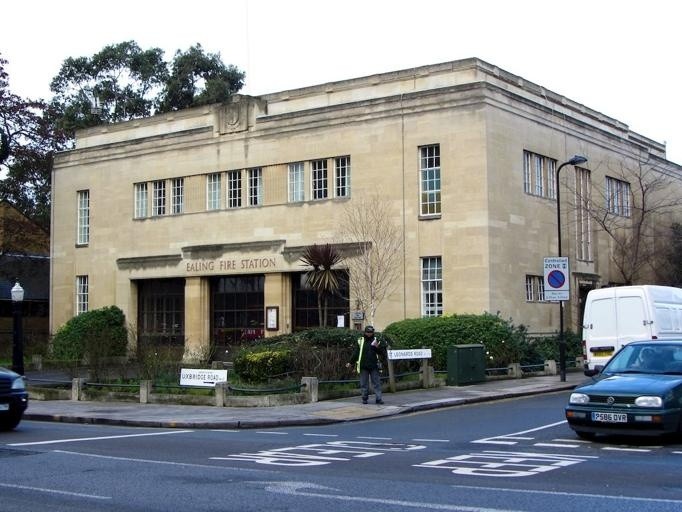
[365, 326, 375, 332]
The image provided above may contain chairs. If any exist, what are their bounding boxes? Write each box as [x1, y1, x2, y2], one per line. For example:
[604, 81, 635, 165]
[639, 348, 654, 366]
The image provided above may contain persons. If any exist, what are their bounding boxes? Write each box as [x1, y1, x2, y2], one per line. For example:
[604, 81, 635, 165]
[345, 325, 388, 404]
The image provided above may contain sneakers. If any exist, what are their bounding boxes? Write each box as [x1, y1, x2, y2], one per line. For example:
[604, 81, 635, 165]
[376, 400, 384, 404]
[363, 400, 368, 404]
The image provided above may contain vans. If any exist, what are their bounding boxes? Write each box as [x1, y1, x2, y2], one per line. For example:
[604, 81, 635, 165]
[582, 284, 681, 378]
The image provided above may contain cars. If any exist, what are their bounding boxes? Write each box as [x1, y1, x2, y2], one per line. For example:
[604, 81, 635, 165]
[0, 366, 28, 431]
[563, 338, 681, 445]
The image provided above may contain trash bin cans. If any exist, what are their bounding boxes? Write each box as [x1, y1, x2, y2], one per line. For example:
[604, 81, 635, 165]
[447, 343, 484, 386]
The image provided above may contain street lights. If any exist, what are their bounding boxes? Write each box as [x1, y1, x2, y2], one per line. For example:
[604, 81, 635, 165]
[9, 277, 25, 376]
[554, 156, 588, 381]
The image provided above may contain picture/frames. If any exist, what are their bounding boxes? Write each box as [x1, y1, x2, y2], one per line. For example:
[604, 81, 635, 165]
[265, 305, 277, 331]
